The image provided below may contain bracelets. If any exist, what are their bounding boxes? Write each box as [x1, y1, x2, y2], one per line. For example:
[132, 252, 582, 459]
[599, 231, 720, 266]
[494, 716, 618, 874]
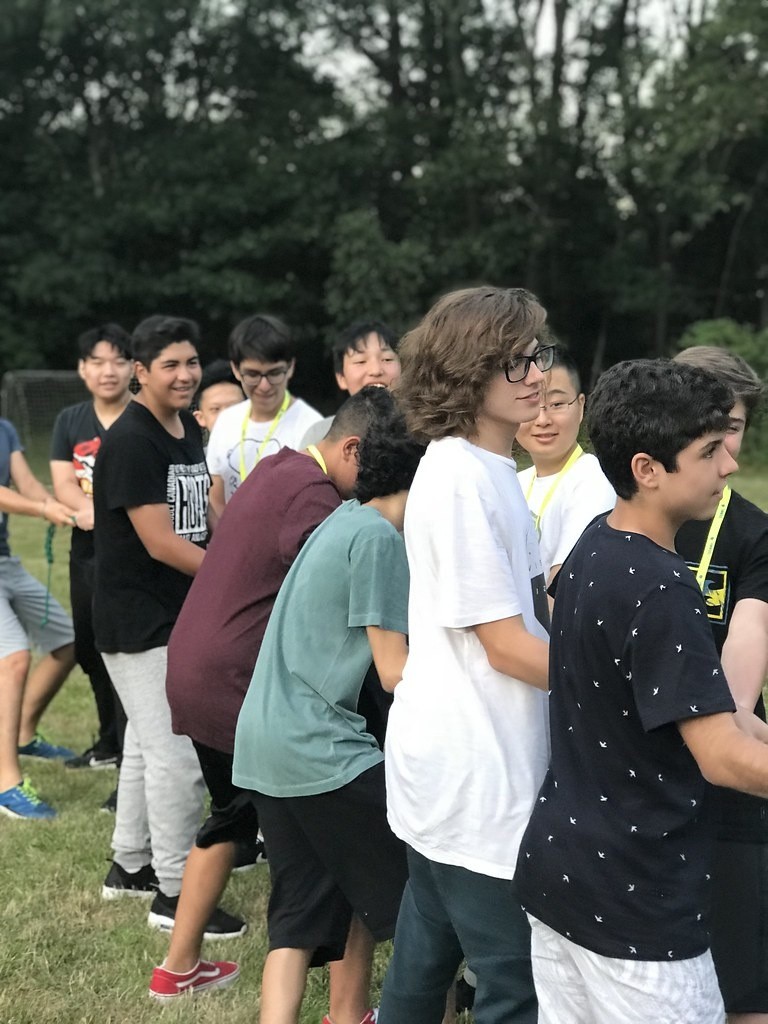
[41, 503, 48, 520]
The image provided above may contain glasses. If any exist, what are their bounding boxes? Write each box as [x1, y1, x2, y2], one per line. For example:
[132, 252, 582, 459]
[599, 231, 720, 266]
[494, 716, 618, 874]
[540, 394, 581, 413]
[496, 343, 557, 384]
[234, 366, 293, 386]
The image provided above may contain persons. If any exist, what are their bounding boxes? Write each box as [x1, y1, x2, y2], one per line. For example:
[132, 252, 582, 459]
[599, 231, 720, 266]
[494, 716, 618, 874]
[51, 288, 768, 1024]
[0, 418, 80, 818]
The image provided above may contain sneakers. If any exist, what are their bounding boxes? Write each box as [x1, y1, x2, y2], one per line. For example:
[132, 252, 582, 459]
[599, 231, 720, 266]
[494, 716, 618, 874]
[102, 788, 118, 814]
[321, 1007, 379, 1024]
[17, 732, 75, 763]
[149, 957, 242, 1002]
[63, 747, 119, 771]
[147, 883, 248, 938]
[0, 775, 53, 821]
[228, 835, 270, 873]
[102, 857, 160, 899]
